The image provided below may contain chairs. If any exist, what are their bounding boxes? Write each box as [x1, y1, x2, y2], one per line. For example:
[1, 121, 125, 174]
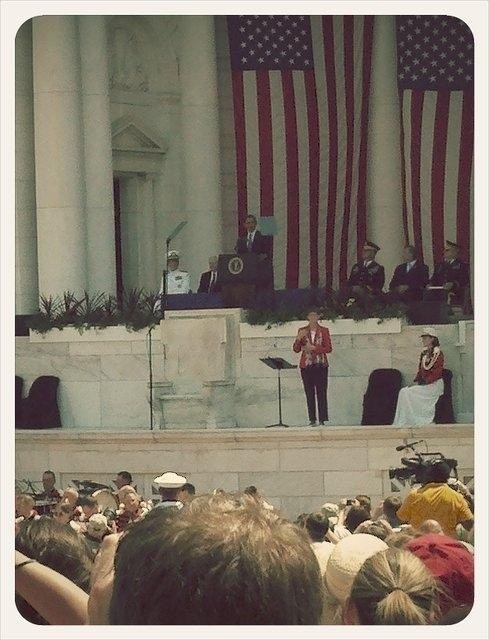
[16, 377, 24, 402]
[16, 375, 60, 430]
[418, 369, 454, 424]
[361, 369, 402, 425]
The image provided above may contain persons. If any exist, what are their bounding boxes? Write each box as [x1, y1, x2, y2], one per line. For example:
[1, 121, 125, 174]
[347, 237, 386, 293]
[234, 214, 267, 255]
[15, 463, 474, 625]
[153, 249, 191, 311]
[426, 240, 470, 306]
[289, 307, 335, 427]
[390, 326, 445, 428]
[390, 244, 430, 292]
[197, 254, 220, 294]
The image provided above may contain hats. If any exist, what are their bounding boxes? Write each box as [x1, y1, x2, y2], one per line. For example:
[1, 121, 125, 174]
[420, 327, 438, 339]
[322, 532, 390, 608]
[442, 240, 462, 251]
[165, 250, 182, 260]
[154, 471, 187, 489]
[360, 240, 380, 252]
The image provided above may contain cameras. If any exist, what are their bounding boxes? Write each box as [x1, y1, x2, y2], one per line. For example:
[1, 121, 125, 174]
[346, 500, 355, 506]
[103, 508, 116, 527]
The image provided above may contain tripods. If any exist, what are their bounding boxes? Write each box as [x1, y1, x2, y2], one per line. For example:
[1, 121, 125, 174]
[265, 370, 290, 429]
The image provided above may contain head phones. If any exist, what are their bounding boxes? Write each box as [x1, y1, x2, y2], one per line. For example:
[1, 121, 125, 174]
[430, 460, 442, 479]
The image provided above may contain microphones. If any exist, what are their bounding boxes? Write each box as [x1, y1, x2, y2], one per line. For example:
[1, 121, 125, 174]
[146, 323, 156, 335]
[246, 240, 250, 249]
[396, 440, 422, 451]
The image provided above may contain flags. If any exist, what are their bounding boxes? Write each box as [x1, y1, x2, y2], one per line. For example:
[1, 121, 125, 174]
[394, 15, 476, 282]
[222, 17, 376, 296]
